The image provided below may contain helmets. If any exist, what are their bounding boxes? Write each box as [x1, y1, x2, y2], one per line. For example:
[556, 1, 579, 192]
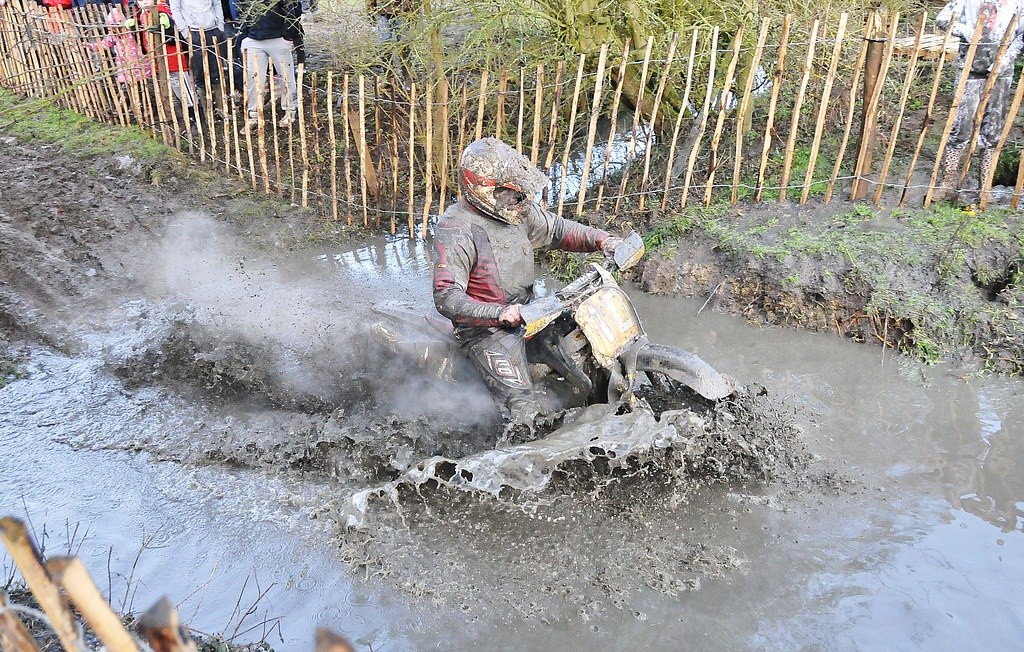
[459, 137, 549, 224]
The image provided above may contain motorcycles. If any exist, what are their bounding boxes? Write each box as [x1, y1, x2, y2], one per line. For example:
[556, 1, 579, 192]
[372, 233, 734, 421]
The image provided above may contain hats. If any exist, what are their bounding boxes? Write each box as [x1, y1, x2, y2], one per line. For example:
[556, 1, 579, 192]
[108, 8, 124, 24]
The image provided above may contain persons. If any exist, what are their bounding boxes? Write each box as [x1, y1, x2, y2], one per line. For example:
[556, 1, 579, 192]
[923, 0, 1024, 203]
[433, 136, 625, 450]
[0, 0, 318, 136]
[364, 0, 422, 88]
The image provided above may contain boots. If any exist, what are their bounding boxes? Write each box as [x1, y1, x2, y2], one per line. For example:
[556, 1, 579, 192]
[264, 75, 281, 111]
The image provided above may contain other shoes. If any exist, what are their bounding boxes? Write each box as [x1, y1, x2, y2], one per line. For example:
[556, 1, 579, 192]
[402, 79, 411, 91]
[381, 79, 396, 88]
[191, 123, 208, 136]
[215, 113, 231, 125]
[296, 66, 306, 74]
[278, 111, 296, 128]
[240, 112, 259, 135]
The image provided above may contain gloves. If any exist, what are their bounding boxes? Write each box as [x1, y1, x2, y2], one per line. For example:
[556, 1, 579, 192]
[160, 13, 170, 29]
[125, 19, 135, 30]
[603, 238, 624, 258]
